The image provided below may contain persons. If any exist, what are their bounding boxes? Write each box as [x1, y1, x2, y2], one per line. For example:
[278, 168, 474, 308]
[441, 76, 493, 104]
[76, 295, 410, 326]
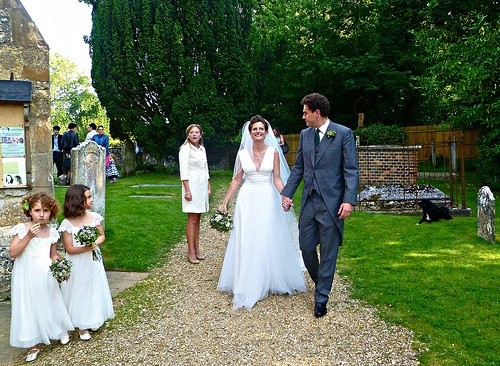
[56, 185, 115, 340]
[61, 123, 80, 184]
[218, 115, 309, 309]
[178, 124, 212, 264]
[106, 153, 120, 183]
[8, 192, 75, 362]
[273, 127, 285, 154]
[280, 92, 358, 318]
[85, 123, 99, 141]
[92, 126, 109, 157]
[52, 126, 62, 177]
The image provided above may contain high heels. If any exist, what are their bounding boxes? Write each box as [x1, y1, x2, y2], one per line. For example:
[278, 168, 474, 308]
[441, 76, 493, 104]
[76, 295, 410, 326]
[187, 253, 199, 264]
[196, 252, 205, 260]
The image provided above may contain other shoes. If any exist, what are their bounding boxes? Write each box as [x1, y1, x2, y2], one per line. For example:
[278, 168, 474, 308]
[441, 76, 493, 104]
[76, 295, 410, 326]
[60, 333, 70, 344]
[26, 349, 40, 361]
[79, 330, 91, 340]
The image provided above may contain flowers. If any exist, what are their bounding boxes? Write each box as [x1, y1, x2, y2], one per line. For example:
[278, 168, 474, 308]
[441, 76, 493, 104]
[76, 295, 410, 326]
[47, 252, 74, 285]
[73, 223, 104, 262]
[326, 129, 338, 139]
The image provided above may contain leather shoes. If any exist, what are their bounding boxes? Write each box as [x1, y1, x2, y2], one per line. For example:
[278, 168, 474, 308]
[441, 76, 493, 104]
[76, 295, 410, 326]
[315, 301, 327, 317]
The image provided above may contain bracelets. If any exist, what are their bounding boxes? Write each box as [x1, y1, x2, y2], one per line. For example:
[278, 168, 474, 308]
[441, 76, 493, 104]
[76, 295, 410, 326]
[185, 192, 191, 195]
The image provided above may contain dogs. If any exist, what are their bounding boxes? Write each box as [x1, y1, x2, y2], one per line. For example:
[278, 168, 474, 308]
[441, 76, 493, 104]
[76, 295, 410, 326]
[415, 199, 452, 226]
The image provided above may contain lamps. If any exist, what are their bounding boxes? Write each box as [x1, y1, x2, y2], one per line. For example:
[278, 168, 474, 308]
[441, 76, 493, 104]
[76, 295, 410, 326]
[207, 198, 235, 232]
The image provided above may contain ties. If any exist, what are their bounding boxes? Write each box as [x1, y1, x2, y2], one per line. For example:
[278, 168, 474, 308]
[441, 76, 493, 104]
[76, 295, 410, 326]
[315, 129, 320, 146]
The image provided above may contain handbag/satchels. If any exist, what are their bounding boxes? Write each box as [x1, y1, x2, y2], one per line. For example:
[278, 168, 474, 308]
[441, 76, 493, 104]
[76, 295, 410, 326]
[282, 142, 289, 154]
